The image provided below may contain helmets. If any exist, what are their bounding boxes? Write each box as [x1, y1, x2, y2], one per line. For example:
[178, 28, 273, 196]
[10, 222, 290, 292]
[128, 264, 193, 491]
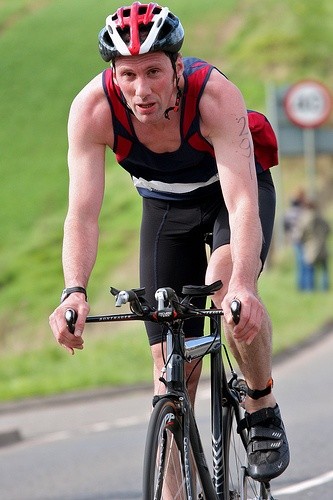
[97, 1, 186, 62]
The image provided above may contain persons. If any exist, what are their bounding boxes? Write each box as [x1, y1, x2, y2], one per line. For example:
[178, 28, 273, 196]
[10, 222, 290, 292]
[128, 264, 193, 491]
[49, 2, 289, 500]
[282, 194, 331, 294]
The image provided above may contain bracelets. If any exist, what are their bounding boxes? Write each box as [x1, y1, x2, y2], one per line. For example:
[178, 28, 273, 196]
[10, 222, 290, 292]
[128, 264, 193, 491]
[60, 286, 88, 302]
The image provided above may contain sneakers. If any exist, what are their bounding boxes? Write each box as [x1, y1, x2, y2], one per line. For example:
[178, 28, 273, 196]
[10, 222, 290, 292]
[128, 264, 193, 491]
[242, 405, 290, 482]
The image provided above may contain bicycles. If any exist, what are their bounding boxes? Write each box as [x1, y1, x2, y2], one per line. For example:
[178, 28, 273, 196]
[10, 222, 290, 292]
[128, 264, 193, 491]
[64, 231, 274, 500]
